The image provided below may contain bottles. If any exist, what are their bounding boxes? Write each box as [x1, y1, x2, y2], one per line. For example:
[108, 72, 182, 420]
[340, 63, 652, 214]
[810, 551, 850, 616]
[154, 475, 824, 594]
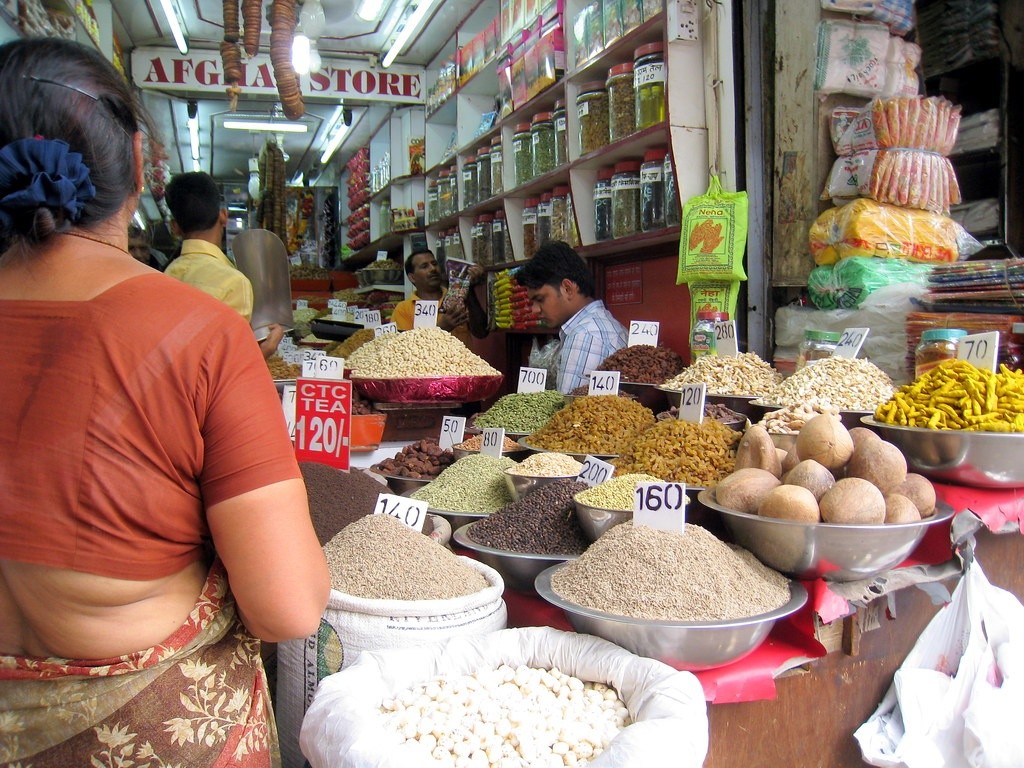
[430, 43, 681, 275]
[794, 331, 840, 374]
[913, 329, 967, 379]
[689, 311, 732, 366]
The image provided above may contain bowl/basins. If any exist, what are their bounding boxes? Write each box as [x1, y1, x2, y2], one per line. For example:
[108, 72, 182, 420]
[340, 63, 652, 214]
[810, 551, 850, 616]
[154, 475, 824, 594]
[299, 319, 365, 350]
[355, 269, 404, 287]
[271, 365, 1024, 673]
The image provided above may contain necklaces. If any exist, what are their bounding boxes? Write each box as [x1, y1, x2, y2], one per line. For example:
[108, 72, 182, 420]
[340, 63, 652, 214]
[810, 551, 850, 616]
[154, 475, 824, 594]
[63, 231, 129, 254]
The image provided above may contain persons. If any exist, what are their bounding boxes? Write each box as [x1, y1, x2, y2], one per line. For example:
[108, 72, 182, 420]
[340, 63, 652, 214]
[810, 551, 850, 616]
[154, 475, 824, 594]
[391, 249, 489, 352]
[514, 241, 629, 394]
[128, 222, 174, 270]
[0, 36, 332, 768]
[163, 172, 283, 357]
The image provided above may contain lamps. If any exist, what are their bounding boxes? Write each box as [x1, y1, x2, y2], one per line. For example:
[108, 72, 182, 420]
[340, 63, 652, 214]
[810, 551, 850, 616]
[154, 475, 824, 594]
[189, 113, 199, 159]
[160, 0, 188, 54]
[223, 122, 307, 132]
[309, 44, 321, 73]
[382, 0, 434, 67]
[356, 0, 384, 21]
[248, 159, 260, 199]
[320, 122, 349, 163]
[291, 34, 310, 75]
[301, 1, 324, 39]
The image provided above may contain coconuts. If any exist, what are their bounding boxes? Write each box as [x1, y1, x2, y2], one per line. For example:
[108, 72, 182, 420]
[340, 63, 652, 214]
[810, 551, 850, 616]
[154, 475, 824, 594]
[717, 414, 936, 526]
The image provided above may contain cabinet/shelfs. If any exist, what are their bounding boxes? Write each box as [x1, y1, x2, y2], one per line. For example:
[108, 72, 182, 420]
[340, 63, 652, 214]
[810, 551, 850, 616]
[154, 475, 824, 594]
[339, 105, 425, 263]
[425, 0, 709, 272]
